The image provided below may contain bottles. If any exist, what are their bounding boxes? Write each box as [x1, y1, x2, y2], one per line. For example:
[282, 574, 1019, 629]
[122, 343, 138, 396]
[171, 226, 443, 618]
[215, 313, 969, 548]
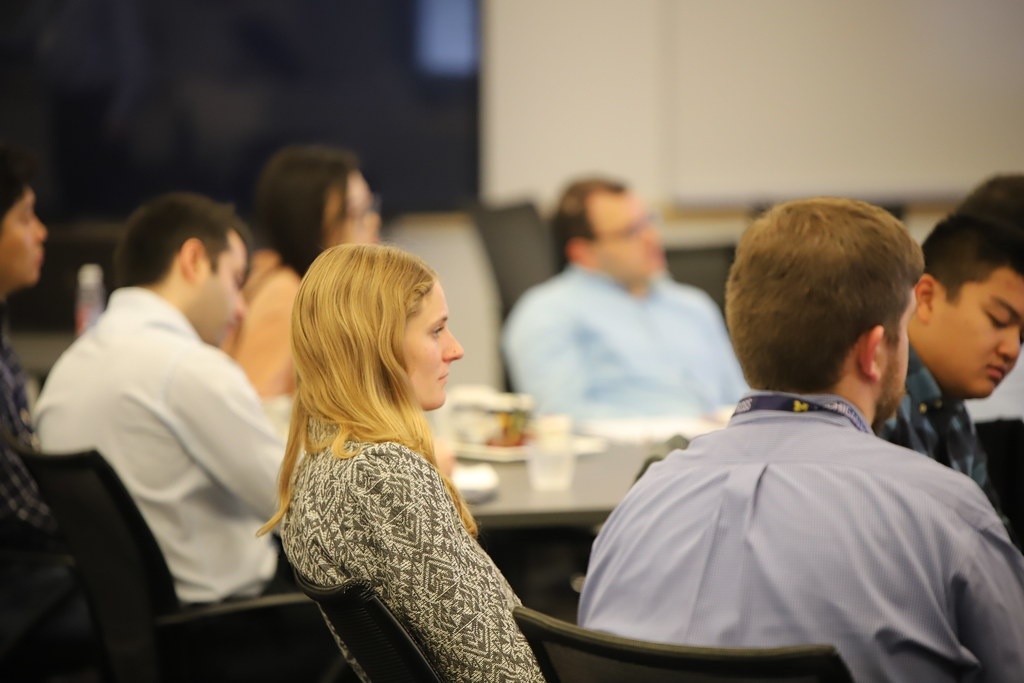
[77, 265, 103, 337]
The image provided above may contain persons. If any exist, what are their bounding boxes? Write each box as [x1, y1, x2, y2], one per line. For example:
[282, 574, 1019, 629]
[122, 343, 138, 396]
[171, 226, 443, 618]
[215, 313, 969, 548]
[873, 171, 1024, 535]
[220, 141, 384, 395]
[574, 198, 1024, 683]
[249, 243, 547, 682]
[498, 176, 750, 423]
[0, 147, 59, 539]
[25, 187, 289, 607]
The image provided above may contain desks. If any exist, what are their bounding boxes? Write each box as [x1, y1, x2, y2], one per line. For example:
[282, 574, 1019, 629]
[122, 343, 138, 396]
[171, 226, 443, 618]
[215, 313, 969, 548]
[261, 398, 734, 530]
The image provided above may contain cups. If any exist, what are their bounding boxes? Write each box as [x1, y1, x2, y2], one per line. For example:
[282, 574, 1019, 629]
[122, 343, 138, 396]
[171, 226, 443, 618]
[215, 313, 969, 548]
[527, 435, 572, 493]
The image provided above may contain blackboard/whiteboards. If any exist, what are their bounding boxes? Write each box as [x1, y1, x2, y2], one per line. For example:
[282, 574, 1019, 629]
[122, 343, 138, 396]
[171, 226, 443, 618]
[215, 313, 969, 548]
[478, 3, 1024, 196]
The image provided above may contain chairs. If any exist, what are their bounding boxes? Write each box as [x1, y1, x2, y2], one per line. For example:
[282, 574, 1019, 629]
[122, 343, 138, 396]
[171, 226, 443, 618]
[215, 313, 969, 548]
[0, 200, 1024, 683]
